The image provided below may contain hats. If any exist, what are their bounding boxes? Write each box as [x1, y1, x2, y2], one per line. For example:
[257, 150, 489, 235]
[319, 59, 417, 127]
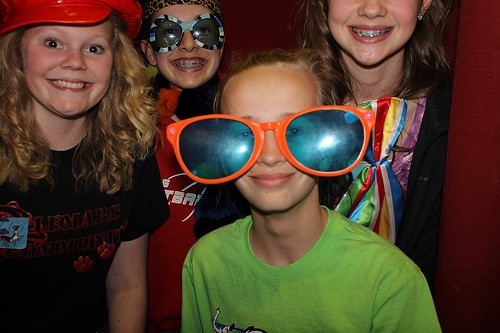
[0, 0, 142, 42]
[144, 0, 222, 15]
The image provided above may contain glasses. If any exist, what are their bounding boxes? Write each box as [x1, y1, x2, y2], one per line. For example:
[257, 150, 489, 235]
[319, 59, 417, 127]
[165, 106, 375, 183]
[146, 12, 224, 53]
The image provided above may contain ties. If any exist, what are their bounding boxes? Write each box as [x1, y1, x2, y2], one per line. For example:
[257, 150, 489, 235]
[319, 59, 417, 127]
[335, 96, 404, 246]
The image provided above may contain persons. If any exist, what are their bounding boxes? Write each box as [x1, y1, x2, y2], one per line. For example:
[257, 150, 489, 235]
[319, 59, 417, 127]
[182, 50, 443, 332]
[137, 1, 229, 332]
[297, 0, 454, 297]
[1, 1, 169, 333]
